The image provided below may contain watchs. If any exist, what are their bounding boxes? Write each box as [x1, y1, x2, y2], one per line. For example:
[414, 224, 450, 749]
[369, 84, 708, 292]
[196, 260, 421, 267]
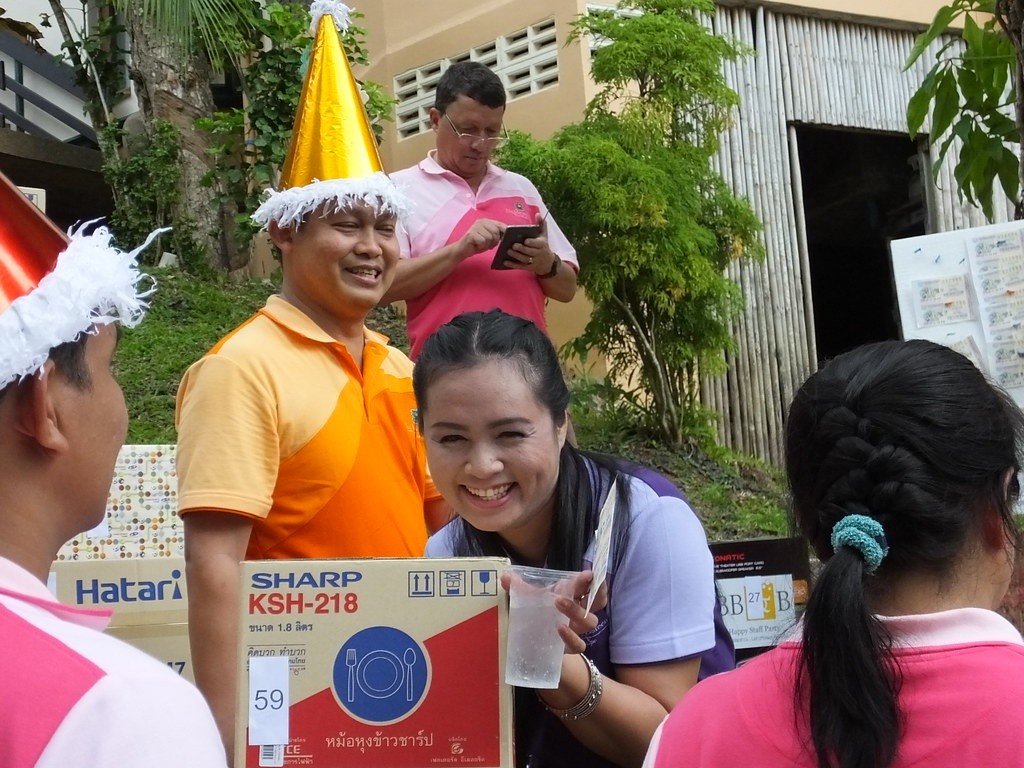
[535, 252, 561, 279]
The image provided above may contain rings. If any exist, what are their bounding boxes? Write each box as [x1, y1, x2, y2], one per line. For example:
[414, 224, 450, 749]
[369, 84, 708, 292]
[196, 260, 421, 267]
[527, 257, 533, 265]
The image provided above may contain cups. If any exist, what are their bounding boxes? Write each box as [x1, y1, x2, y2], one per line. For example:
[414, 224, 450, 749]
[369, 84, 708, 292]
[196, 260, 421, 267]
[505, 565, 582, 689]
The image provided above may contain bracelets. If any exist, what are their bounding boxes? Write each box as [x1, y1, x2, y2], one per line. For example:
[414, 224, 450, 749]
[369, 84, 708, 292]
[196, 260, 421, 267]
[535, 653, 603, 721]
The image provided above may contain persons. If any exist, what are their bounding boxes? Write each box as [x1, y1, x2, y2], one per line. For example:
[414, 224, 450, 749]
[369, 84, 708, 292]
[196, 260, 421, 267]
[379, 62, 580, 361]
[175, 196, 458, 768]
[0, 173, 229, 768]
[413, 306, 737, 768]
[642, 340, 1024, 768]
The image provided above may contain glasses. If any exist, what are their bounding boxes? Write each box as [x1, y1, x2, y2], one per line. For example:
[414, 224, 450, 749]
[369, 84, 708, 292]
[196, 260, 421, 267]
[439, 108, 510, 150]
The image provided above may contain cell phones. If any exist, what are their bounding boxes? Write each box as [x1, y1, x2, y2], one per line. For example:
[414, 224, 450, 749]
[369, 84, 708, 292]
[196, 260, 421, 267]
[492, 223, 540, 271]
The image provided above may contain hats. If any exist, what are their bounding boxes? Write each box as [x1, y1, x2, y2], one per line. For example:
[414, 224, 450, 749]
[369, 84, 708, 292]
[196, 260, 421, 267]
[0, 172, 171, 389]
[250, 0, 417, 231]
